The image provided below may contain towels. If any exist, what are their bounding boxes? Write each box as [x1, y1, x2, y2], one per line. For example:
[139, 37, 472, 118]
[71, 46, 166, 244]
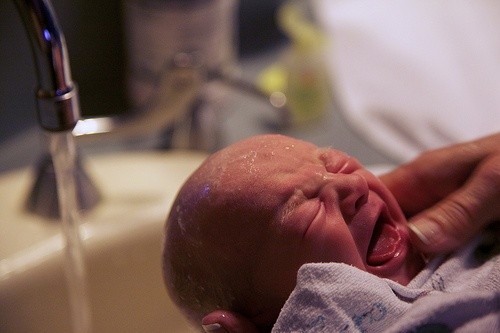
[270, 241, 496, 333]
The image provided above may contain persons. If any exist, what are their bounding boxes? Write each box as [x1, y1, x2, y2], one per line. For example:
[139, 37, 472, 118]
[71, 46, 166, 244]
[201, 130, 500, 332]
[161, 133, 423, 332]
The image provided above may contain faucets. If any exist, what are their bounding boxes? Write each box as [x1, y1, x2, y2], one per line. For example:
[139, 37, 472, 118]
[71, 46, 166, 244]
[8, 0, 80, 134]
